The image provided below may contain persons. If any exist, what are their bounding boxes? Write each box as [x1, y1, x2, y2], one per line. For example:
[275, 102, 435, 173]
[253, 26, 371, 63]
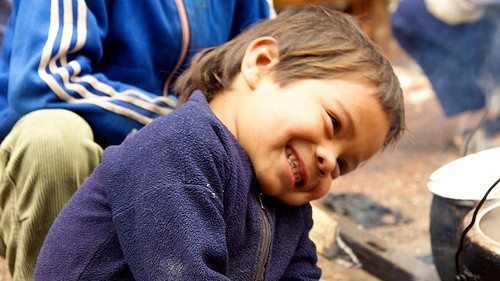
[32, 2, 408, 280]
[0, 0, 278, 281]
